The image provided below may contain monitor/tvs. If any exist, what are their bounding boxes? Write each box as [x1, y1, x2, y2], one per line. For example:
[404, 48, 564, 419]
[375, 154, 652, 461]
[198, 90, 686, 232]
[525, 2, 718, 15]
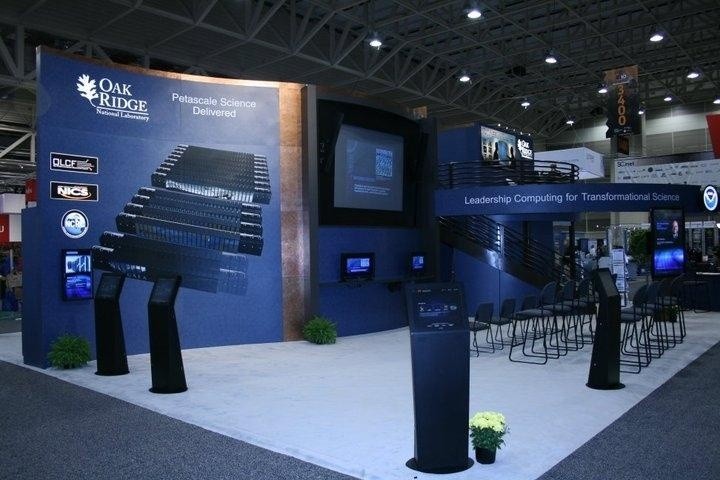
[410, 251, 426, 272]
[341, 251, 377, 280]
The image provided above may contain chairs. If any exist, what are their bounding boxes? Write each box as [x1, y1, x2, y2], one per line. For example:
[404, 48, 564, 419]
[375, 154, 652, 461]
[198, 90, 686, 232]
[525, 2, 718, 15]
[470, 274, 712, 374]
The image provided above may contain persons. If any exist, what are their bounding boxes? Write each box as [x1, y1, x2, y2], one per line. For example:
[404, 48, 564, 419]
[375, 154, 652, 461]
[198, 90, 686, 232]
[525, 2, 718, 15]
[590, 244, 596, 256]
[558, 239, 569, 266]
[669, 220, 680, 245]
[506, 147, 516, 169]
[493, 142, 499, 161]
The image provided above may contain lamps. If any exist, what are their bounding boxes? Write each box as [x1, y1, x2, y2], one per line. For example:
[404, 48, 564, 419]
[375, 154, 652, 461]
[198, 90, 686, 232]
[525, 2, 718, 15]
[684, 69, 700, 81]
[637, 107, 646, 116]
[713, 97, 720, 105]
[662, 94, 672, 102]
[648, 31, 664, 43]
[565, 118, 575, 125]
[519, 97, 533, 110]
[463, 2, 483, 19]
[543, 50, 558, 64]
[596, 83, 608, 95]
[458, 68, 471, 83]
[370, 31, 383, 49]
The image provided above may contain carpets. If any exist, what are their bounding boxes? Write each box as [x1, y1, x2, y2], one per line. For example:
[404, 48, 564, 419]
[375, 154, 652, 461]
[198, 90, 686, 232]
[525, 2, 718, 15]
[1, 309, 720, 479]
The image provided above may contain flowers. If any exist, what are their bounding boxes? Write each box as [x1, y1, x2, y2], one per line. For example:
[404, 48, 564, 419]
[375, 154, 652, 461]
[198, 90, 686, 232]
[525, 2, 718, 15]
[467, 412, 509, 452]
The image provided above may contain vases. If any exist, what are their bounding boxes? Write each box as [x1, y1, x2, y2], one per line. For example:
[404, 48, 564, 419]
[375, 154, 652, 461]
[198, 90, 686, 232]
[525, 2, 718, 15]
[475, 444, 497, 464]
